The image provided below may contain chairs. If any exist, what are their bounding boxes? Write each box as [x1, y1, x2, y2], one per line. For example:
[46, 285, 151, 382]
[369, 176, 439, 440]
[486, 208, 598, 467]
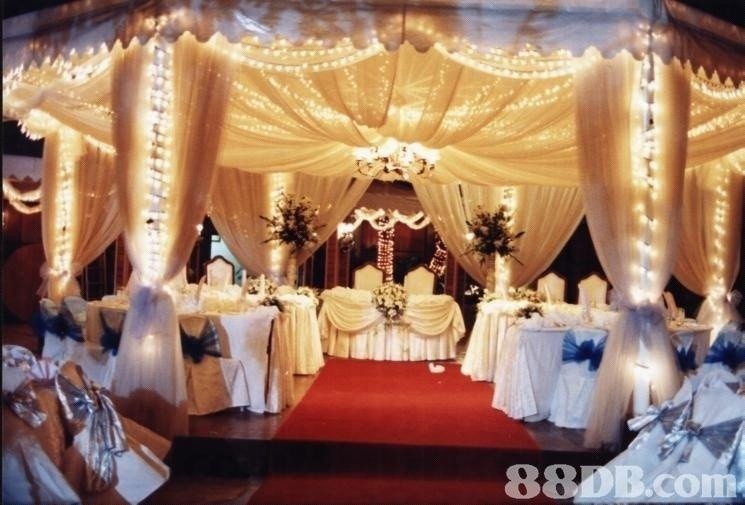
[0, 256, 745, 505]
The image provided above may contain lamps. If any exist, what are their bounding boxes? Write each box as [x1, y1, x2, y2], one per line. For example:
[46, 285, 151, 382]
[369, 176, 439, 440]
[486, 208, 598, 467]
[351, 135, 441, 181]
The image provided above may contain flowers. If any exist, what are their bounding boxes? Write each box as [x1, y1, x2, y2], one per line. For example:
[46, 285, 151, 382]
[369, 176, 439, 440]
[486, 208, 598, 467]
[460, 204, 527, 267]
[259, 190, 332, 257]
